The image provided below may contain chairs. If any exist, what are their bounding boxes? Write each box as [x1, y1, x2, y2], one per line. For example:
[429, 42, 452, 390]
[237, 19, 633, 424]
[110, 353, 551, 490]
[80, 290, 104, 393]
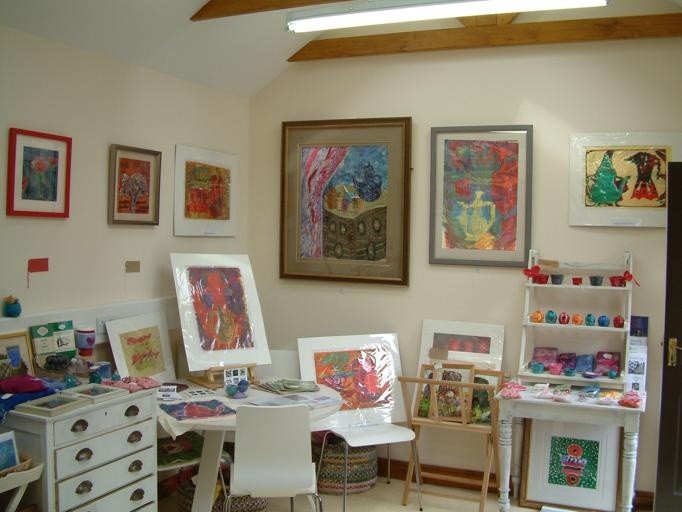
[317, 420, 424, 511]
[218, 397, 329, 512]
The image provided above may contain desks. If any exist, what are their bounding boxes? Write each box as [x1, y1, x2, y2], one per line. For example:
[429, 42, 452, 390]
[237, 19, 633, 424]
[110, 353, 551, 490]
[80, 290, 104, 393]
[161, 366, 350, 512]
[495, 378, 652, 512]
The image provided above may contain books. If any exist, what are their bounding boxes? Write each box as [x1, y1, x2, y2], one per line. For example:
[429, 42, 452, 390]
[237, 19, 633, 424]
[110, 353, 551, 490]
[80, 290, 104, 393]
[250, 376, 321, 397]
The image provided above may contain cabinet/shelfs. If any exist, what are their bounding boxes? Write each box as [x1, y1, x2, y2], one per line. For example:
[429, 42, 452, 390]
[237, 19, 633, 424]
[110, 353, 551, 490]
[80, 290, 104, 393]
[0, 459, 46, 512]
[396, 371, 504, 511]
[514, 251, 636, 392]
[2, 386, 165, 512]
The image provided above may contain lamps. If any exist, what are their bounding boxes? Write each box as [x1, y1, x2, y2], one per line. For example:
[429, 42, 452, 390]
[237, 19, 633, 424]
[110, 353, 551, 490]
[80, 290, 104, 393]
[285, 1, 611, 38]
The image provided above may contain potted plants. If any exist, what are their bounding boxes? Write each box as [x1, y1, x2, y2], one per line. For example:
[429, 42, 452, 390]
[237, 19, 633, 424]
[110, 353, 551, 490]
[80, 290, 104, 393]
[1, 291, 22, 319]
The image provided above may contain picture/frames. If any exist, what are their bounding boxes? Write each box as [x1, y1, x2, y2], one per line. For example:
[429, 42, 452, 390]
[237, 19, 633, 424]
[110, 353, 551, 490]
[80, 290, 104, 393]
[278, 116, 414, 288]
[0, 331, 39, 382]
[568, 133, 678, 234]
[513, 408, 627, 512]
[171, 144, 241, 240]
[105, 142, 162, 227]
[296, 313, 510, 434]
[167, 249, 274, 371]
[5, 126, 75, 220]
[0, 430, 19, 470]
[101, 310, 179, 388]
[431, 126, 536, 270]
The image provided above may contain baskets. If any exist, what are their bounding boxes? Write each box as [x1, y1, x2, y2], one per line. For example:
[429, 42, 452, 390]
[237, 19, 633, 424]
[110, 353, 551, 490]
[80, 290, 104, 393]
[1, 454, 31, 476]
[178, 474, 269, 511]
[313, 439, 379, 495]
